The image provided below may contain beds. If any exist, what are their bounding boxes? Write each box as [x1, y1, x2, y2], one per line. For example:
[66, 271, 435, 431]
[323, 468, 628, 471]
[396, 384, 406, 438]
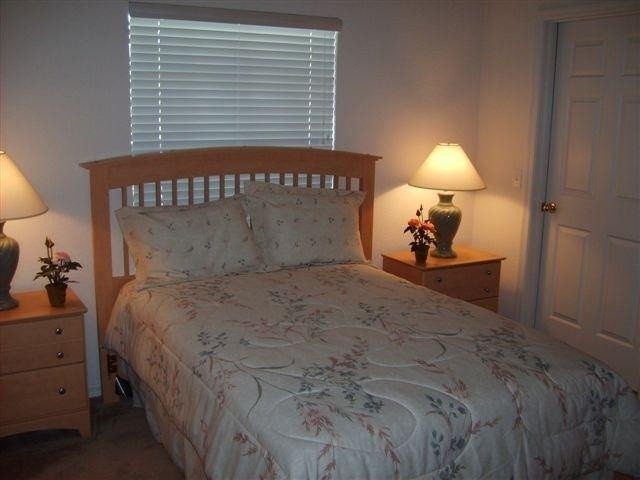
[80, 147, 640, 478]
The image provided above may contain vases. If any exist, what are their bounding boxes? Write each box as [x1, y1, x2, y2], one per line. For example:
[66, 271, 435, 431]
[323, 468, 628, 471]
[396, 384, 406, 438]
[44, 283, 68, 304]
[413, 245, 429, 264]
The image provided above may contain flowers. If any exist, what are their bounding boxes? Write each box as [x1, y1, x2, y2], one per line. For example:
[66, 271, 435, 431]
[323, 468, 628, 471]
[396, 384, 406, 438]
[403, 203, 439, 253]
[33, 237, 82, 287]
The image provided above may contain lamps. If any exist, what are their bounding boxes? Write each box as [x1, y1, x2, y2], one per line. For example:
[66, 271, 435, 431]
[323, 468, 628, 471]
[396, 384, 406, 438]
[0, 150, 50, 310]
[410, 142, 487, 259]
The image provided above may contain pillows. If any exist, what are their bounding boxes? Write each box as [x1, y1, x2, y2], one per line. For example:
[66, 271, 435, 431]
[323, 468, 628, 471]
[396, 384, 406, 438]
[108, 181, 372, 293]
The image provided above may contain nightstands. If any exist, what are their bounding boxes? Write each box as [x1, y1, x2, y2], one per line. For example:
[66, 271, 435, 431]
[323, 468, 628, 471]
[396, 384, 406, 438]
[382, 247, 505, 313]
[1, 289, 96, 441]
